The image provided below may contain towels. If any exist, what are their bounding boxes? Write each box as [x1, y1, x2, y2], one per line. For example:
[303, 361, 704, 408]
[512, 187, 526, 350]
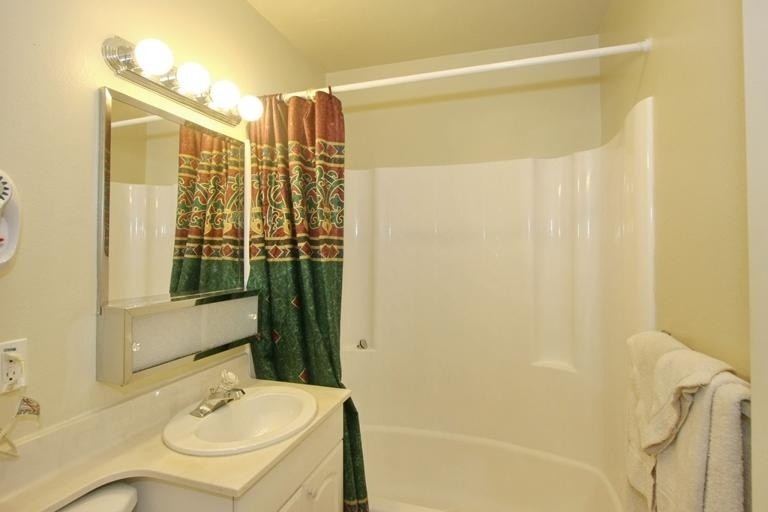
[624, 330, 752, 512]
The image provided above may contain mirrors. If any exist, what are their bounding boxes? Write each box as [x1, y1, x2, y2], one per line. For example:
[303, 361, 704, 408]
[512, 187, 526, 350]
[94, 85, 263, 386]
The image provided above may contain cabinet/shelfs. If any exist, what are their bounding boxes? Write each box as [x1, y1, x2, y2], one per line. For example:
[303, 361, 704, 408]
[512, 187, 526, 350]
[231, 404, 344, 512]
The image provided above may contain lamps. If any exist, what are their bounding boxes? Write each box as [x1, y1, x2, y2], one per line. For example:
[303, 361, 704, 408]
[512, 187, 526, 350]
[100, 35, 264, 129]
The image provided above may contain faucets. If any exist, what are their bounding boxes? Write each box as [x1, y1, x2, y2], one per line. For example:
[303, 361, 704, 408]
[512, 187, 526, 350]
[210, 370, 246, 401]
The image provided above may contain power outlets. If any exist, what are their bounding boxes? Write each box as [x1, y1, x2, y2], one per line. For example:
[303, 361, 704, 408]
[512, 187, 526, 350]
[0, 338, 30, 394]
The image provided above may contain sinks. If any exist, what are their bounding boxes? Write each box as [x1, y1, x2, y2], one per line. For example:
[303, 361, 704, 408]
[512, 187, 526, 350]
[162, 385, 318, 456]
